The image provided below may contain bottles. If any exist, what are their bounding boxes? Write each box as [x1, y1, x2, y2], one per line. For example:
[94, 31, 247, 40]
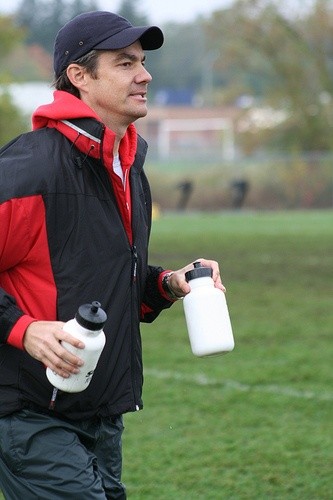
[181, 260, 235, 359]
[46, 299, 109, 394]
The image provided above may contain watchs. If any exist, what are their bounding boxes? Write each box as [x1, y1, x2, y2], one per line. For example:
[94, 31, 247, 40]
[161, 272, 185, 301]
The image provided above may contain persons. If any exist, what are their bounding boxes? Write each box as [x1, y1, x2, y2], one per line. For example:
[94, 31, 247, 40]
[0, 12, 237, 500]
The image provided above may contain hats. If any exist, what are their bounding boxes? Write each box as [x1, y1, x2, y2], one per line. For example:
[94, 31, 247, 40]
[53, 10, 164, 76]
